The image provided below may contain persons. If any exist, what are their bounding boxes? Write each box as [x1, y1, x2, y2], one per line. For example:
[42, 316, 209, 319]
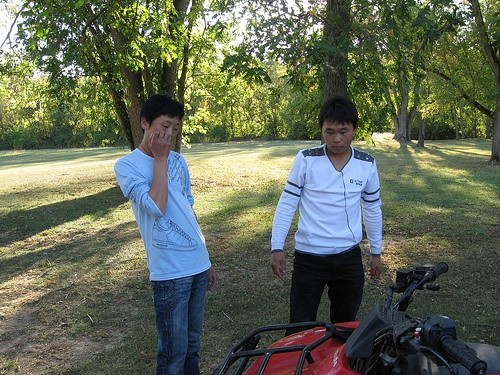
[113, 95, 215, 375]
[271, 96, 382, 338]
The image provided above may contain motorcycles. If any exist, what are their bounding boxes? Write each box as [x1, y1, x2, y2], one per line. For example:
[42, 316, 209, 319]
[210, 263, 500, 374]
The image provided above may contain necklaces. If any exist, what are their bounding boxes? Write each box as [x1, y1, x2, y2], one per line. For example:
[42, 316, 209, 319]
[140, 143, 147, 153]
